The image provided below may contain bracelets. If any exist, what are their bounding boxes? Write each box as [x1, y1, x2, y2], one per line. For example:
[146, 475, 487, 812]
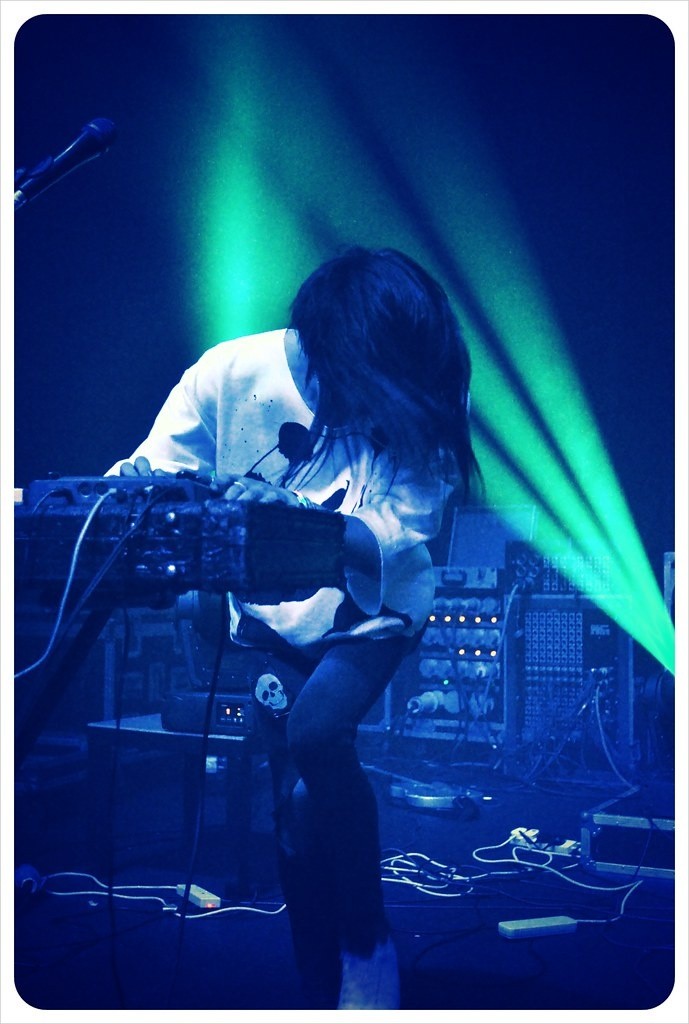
[290, 489, 316, 509]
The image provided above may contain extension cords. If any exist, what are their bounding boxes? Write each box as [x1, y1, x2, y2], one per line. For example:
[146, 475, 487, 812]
[176, 884, 221, 908]
[497, 915, 578, 940]
[509, 826, 578, 854]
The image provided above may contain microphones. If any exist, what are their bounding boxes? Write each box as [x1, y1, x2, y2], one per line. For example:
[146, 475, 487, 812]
[14, 116, 119, 214]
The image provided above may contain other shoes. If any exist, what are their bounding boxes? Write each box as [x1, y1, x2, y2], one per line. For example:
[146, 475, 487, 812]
[337, 928, 402, 1009]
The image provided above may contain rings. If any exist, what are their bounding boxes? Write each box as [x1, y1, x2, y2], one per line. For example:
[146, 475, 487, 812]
[232, 480, 247, 492]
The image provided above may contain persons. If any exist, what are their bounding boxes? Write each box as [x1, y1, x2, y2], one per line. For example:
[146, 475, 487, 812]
[100, 242, 488, 1011]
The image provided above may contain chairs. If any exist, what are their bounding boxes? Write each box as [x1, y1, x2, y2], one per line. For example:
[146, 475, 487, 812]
[85, 596, 286, 898]
